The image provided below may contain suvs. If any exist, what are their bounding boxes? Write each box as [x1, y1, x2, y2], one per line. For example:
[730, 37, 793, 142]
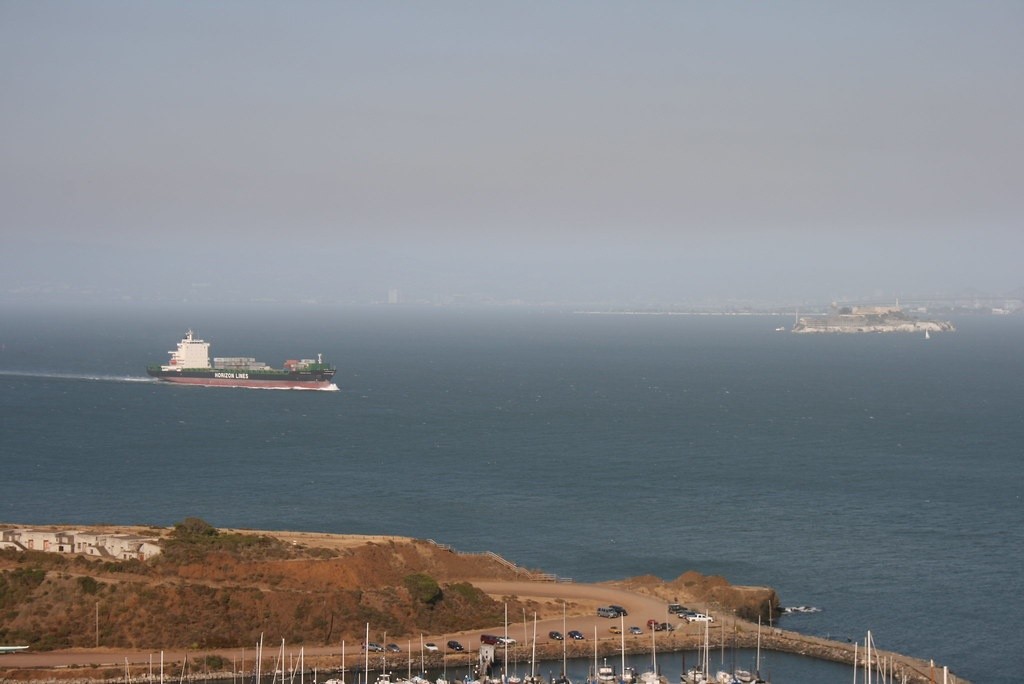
[668, 604, 688, 613]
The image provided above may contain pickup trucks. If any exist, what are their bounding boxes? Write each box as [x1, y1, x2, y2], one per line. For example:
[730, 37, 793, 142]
[686, 613, 714, 624]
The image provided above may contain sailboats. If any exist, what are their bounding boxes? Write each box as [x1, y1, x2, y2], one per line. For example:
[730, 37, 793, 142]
[121, 599, 954, 684]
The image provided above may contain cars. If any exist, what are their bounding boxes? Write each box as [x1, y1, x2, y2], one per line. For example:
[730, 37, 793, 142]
[385, 643, 402, 653]
[549, 630, 564, 640]
[367, 642, 384, 652]
[447, 640, 464, 651]
[678, 611, 696, 618]
[609, 625, 621, 634]
[424, 642, 438, 652]
[568, 630, 584, 640]
[647, 619, 672, 630]
[629, 625, 643, 635]
[496, 637, 517, 645]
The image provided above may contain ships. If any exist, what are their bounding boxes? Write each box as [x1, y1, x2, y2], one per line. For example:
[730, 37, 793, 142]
[146, 327, 338, 388]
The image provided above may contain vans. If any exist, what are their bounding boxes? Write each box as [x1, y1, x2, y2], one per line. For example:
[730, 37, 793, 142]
[481, 634, 503, 645]
[608, 605, 627, 617]
[597, 607, 618, 619]
